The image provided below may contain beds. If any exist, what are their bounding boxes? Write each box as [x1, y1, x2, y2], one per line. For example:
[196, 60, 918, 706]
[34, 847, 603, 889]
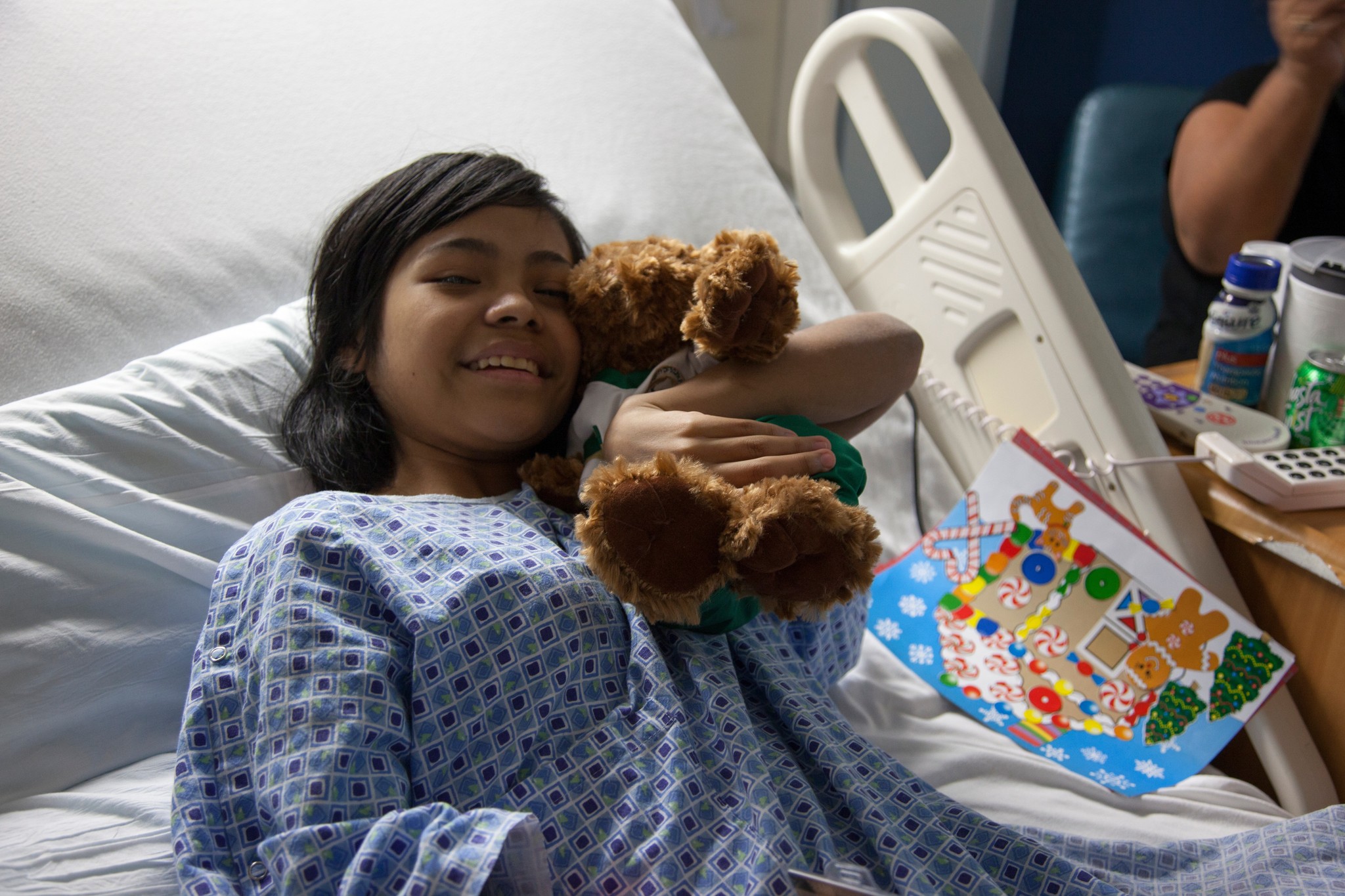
[0, 1, 1341, 896]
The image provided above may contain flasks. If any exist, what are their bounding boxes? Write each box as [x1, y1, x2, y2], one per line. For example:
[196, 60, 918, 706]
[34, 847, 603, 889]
[1266, 235, 1345, 445]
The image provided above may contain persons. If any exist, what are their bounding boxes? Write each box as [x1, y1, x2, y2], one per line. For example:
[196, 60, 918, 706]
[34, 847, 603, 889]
[1145, 0, 1344, 373]
[168, 149, 1345, 896]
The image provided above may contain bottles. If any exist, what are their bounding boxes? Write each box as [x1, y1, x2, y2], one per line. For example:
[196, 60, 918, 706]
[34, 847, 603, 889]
[1197, 254, 1280, 414]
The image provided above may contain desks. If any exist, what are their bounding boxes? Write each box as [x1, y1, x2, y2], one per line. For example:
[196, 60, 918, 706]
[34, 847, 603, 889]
[1138, 353, 1345, 819]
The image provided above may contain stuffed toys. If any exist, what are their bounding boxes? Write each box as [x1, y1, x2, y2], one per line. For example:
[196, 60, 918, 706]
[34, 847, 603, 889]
[524, 230, 881, 629]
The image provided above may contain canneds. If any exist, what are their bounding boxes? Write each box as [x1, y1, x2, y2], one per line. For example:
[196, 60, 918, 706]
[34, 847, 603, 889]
[1281, 350, 1345, 450]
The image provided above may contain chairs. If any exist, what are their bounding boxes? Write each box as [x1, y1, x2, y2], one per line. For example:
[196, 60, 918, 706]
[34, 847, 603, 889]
[1049, 81, 1208, 365]
